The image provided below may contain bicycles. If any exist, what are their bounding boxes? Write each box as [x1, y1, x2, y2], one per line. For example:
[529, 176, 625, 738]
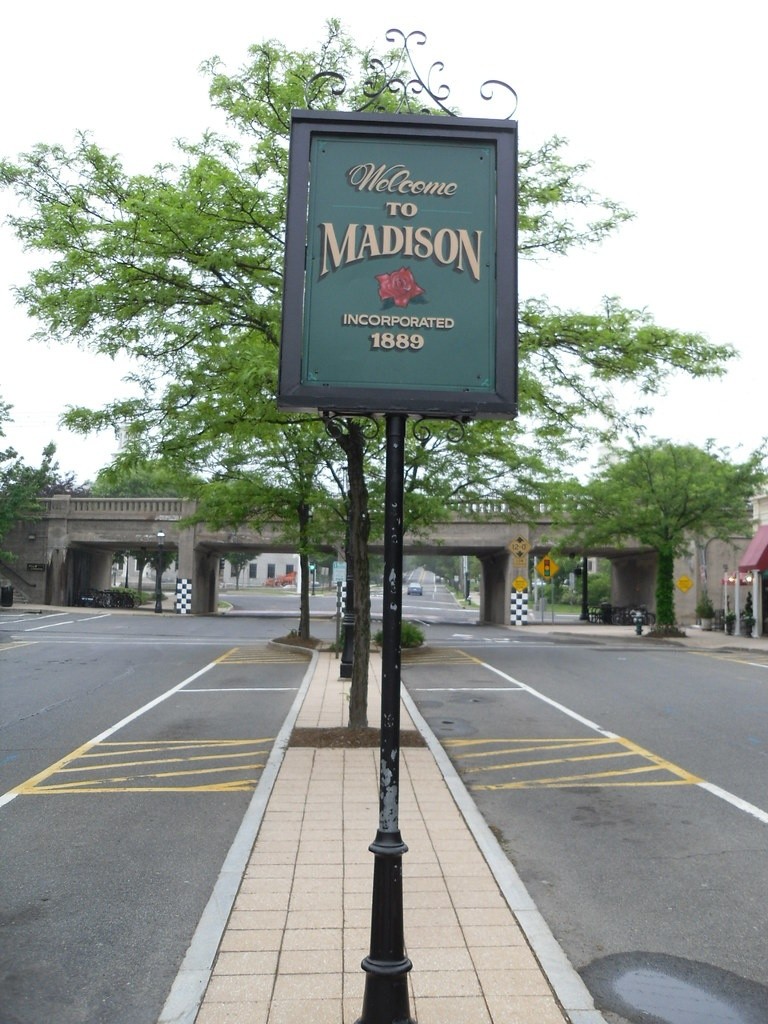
[586, 602, 656, 626]
[71, 586, 142, 609]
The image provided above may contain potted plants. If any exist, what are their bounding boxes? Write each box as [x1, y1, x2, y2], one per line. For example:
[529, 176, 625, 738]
[741, 615, 756, 638]
[694, 598, 716, 631]
[720, 611, 736, 636]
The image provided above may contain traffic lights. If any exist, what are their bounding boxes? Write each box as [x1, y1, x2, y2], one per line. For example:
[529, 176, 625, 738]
[310, 561, 315, 574]
[544, 559, 550, 576]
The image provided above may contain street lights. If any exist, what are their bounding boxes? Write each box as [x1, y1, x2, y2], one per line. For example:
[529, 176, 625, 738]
[155, 529, 166, 613]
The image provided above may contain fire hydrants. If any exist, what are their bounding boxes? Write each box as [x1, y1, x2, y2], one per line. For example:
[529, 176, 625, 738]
[632, 609, 646, 634]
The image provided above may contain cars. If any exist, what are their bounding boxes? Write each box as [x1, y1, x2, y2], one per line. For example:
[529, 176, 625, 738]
[406, 582, 424, 596]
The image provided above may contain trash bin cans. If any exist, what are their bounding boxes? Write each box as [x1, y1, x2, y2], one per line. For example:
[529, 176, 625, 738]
[0, 585, 14, 607]
